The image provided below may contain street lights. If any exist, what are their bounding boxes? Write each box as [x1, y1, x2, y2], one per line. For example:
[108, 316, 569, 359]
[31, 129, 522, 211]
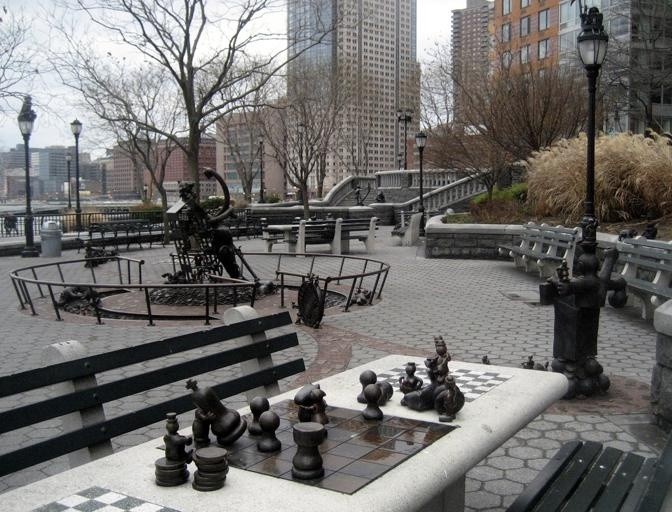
[64, 117, 88, 234]
[575, 5, 610, 260]
[257, 133, 267, 204]
[415, 131, 429, 236]
[395, 107, 414, 170]
[16, 109, 44, 259]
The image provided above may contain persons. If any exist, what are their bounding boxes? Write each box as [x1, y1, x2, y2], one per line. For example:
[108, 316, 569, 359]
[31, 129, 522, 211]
[3, 212, 19, 236]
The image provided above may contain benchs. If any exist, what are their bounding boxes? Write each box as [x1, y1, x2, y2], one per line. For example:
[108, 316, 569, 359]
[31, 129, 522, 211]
[390, 211, 423, 246]
[260, 211, 380, 259]
[498, 221, 581, 281]
[75, 224, 165, 254]
[598, 234, 672, 320]
[0, 304, 305, 481]
[501, 434, 671, 511]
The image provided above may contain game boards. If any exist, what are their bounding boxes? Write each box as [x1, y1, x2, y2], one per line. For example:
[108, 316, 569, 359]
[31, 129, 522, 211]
[28, 486, 184, 512]
[355, 361, 514, 415]
[155, 396, 461, 497]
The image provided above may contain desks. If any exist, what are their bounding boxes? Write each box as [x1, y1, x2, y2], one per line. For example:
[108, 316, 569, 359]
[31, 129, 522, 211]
[0, 354, 570, 511]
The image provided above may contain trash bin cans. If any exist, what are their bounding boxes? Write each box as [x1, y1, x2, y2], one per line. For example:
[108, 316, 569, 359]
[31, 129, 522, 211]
[39, 221, 64, 257]
